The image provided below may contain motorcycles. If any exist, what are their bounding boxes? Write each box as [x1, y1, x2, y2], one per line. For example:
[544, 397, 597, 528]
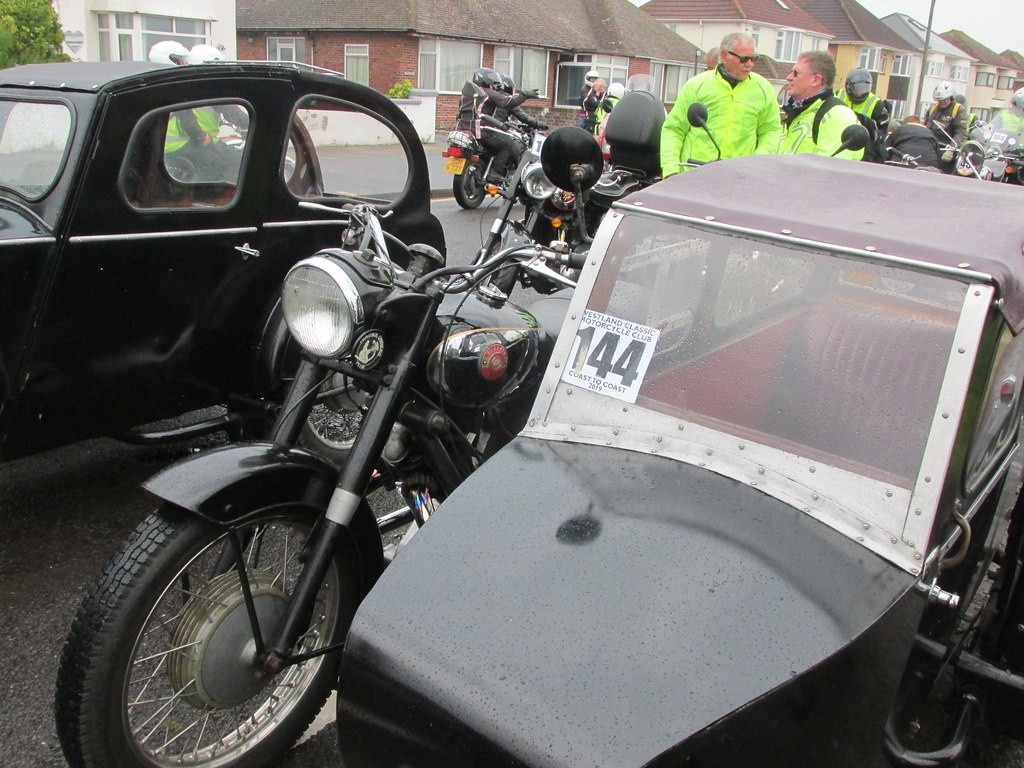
[468, 87, 667, 300]
[166, 123, 295, 183]
[829, 105, 1024, 189]
[441, 106, 552, 210]
[54, 124, 1024, 767]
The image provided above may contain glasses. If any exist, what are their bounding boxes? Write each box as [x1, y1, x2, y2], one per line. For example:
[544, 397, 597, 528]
[791, 69, 812, 77]
[726, 50, 759, 63]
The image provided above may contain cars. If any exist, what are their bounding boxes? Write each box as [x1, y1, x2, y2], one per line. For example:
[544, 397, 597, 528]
[1, 62, 444, 470]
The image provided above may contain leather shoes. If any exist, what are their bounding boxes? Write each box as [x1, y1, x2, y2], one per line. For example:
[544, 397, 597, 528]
[486, 172, 507, 182]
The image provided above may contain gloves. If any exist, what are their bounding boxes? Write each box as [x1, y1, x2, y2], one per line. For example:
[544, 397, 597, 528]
[523, 88, 539, 99]
[199, 132, 227, 155]
[941, 151, 954, 161]
[535, 120, 549, 130]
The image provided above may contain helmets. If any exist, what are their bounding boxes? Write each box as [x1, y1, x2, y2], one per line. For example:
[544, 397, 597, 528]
[1011, 86, 1024, 110]
[933, 80, 958, 101]
[845, 67, 872, 104]
[472, 67, 514, 95]
[584, 70, 599, 88]
[149, 40, 229, 67]
[606, 82, 625, 100]
[955, 94, 967, 110]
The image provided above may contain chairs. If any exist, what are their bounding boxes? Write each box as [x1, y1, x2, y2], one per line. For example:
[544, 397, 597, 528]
[130, 139, 262, 228]
[774, 301, 955, 491]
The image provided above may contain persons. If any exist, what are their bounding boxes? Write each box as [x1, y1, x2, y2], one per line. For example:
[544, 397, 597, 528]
[456, 67, 546, 182]
[885, 115, 940, 169]
[966, 87, 1024, 157]
[578, 70, 624, 137]
[660, 33, 865, 181]
[705, 47, 720, 70]
[148, 40, 250, 184]
[833, 67, 890, 164]
[923, 81, 978, 174]
